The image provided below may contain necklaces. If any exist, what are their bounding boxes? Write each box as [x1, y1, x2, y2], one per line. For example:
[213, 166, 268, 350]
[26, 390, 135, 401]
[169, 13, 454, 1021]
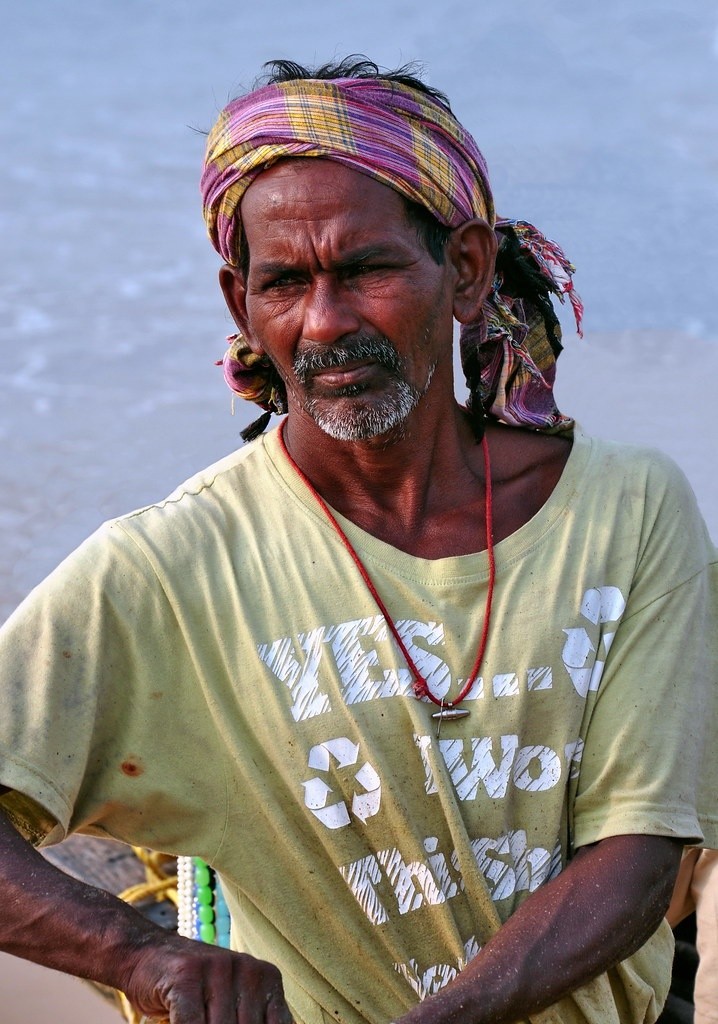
[279, 414, 497, 741]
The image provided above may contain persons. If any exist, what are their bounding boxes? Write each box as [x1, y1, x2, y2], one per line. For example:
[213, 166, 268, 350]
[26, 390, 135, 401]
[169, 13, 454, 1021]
[0, 58, 718, 1024]
